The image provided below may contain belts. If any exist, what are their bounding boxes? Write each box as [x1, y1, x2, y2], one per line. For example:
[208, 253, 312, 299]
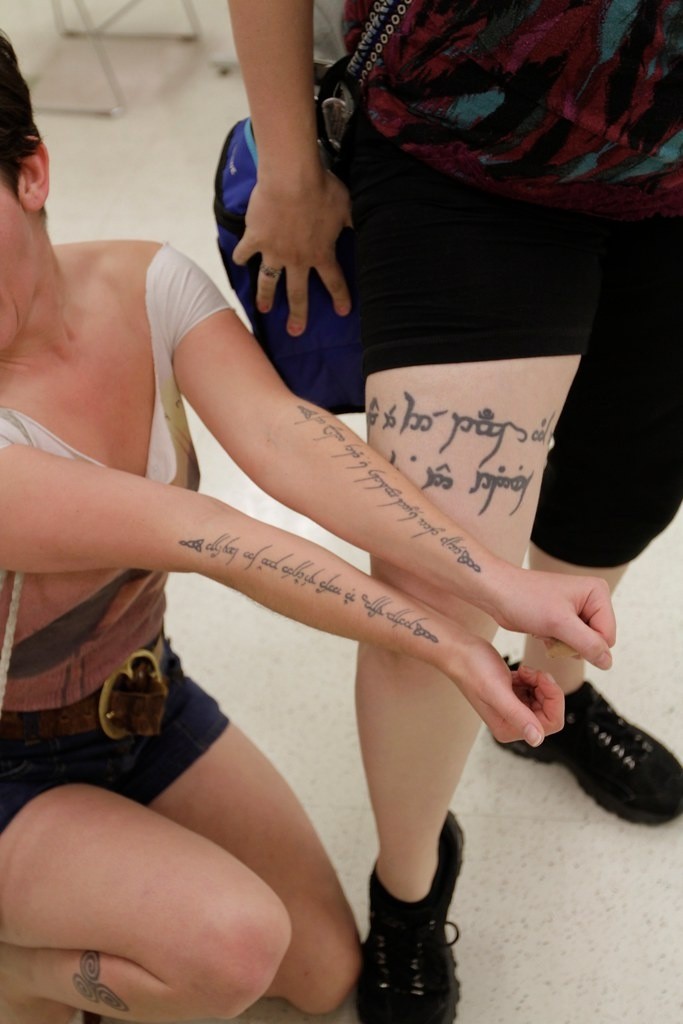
[0, 631, 167, 741]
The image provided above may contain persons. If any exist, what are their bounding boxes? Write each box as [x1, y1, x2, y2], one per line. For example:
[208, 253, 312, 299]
[0, 32, 617, 1024]
[227, 0, 682, 1024]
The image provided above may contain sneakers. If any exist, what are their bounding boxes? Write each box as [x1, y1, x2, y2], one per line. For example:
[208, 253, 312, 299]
[493, 655, 683, 824]
[357, 810, 465, 1024]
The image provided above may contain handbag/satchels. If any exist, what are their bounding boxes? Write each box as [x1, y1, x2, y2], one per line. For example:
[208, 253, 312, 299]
[211, 55, 368, 415]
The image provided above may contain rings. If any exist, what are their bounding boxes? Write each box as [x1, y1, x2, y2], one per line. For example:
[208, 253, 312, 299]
[260, 263, 281, 278]
